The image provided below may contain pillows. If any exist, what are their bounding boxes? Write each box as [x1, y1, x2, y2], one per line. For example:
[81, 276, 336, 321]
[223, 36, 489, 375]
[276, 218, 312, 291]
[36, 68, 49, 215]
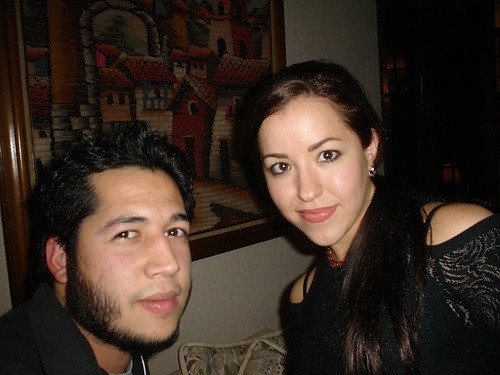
[238, 339, 286, 375]
[177, 329, 288, 375]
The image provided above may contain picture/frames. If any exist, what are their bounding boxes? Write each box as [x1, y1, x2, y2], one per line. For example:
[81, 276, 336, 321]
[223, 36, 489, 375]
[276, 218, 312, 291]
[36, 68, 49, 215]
[0, 0, 286, 309]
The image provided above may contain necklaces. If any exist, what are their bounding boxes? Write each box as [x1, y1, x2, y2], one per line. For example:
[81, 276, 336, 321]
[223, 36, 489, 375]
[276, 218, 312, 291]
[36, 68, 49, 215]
[326, 249, 346, 269]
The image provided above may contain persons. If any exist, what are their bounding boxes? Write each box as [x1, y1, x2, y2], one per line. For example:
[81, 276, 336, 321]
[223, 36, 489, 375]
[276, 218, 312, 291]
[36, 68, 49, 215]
[248, 58, 500, 375]
[0, 121, 196, 375]
